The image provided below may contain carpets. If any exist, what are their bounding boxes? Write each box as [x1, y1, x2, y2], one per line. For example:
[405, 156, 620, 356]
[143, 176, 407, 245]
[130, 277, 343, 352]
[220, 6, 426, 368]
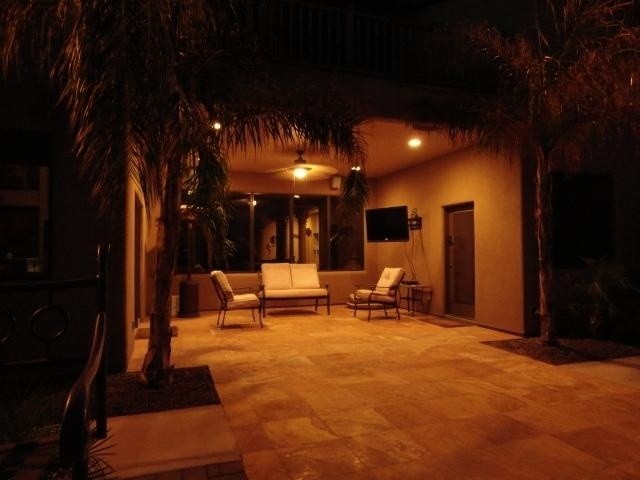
[417, 316, 474, 328]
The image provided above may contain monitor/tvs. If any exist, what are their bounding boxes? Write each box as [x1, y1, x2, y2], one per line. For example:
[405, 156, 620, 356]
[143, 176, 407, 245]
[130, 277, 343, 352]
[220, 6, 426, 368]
[366, 206, 409, 240]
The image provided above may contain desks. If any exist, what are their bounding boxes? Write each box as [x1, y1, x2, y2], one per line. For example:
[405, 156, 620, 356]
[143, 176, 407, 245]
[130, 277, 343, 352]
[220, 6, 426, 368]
[368, 283, 433, 316]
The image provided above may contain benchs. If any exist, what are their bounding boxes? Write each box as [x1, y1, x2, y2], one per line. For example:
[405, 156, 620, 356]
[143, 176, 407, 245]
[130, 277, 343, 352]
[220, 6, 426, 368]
[255, 263, 330, 317]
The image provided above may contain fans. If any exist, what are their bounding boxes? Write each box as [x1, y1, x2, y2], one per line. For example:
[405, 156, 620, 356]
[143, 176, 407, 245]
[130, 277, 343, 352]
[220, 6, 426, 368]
[264, 150, 338, 182]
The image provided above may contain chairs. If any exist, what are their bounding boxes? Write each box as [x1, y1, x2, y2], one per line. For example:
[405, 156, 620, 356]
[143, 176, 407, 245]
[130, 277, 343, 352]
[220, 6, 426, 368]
[346, 266, 405, 321]
[209, 270, 263, 330]
